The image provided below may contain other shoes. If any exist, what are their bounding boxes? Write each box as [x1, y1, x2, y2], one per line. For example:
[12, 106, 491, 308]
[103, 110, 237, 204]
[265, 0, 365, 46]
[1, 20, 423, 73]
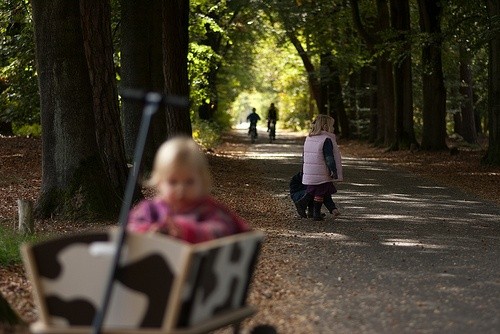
[308, 213, 326, 218]
[297, 207, 307, 218]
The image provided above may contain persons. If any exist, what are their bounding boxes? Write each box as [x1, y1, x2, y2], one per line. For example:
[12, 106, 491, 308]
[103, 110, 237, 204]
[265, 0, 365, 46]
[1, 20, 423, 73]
[302, 114, 343, 220]
[246, 107, 261, 137]
[265, 103, 280, 140]
[126, 135, 248, 245]
[290, 161, 327, 218]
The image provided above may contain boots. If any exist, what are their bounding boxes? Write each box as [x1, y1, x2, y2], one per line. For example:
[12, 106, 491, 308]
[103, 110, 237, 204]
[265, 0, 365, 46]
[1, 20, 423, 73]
[313, 200, 326, 221]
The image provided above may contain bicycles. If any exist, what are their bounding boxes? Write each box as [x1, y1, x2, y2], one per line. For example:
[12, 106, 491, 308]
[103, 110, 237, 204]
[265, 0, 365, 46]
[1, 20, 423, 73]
[266, 117, 279, 143]
[247, 118, 260, 143]
